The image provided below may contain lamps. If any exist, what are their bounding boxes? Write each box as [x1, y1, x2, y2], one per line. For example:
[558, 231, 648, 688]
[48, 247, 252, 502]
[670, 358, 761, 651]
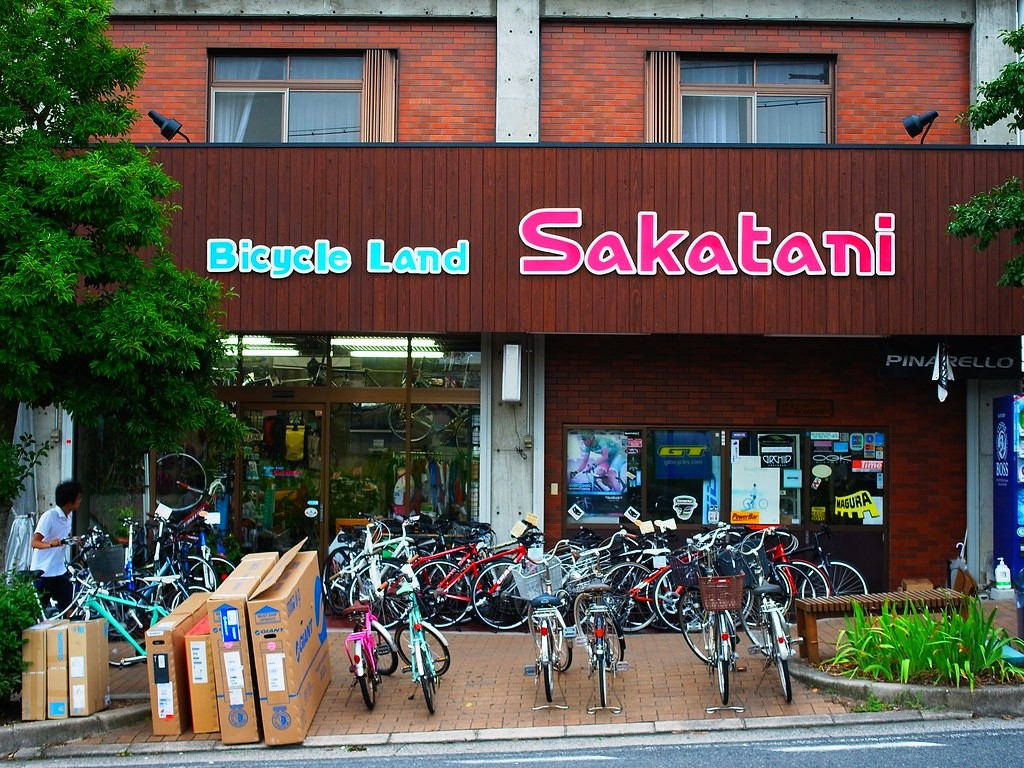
[148, 109, 191, 144]
[902, 109, 939, 144]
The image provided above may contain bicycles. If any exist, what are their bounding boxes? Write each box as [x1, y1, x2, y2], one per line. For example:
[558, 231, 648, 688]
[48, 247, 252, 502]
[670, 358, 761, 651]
[298, 346, 389, 418]
[11, 452, 238, 673]
[666, 527, 747, 714]
[387, 353, 482, 463]
[715, 526, 803, 704]
[326, 550, 400, 709]
[510, 539, 582, 710]
[573, 531, 636, 715]
[324, 516, 869, 633]
[377, 574, 451, 715]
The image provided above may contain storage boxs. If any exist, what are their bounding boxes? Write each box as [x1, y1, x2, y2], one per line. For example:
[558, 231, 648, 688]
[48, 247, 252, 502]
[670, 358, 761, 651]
[144, 537, 333, 747]
[901, 579, 933, 591]
[20, 619, 111, 721]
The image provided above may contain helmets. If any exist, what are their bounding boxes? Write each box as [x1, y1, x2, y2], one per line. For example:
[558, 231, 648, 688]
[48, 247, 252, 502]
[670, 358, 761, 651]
[580, 429, 595, 442]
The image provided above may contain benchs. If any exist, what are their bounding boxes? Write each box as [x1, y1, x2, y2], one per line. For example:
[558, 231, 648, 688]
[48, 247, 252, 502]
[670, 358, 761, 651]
[795, 588, 971, 670]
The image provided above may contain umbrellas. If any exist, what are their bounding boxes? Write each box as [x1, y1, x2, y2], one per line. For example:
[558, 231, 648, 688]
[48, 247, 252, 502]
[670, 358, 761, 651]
[949, 542, 966, 589]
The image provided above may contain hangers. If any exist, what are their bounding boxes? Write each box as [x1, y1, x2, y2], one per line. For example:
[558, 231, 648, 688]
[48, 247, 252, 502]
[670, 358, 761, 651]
[395, 451, 462, 460]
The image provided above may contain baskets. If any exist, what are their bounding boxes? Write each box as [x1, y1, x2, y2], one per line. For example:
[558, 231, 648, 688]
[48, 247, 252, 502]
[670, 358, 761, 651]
[718, 539, 770, 584]
[666, 543, 717, 586]
[560, 549, 612, 591]
[508, 555, 562, 600]
[698, 571, 746, 609]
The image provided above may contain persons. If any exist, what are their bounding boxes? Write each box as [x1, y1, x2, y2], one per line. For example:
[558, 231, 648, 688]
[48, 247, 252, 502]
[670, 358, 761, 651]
[571, 429, 627, 492]
[30, 478, 86, 621]
[392, 467, 414, 515]
